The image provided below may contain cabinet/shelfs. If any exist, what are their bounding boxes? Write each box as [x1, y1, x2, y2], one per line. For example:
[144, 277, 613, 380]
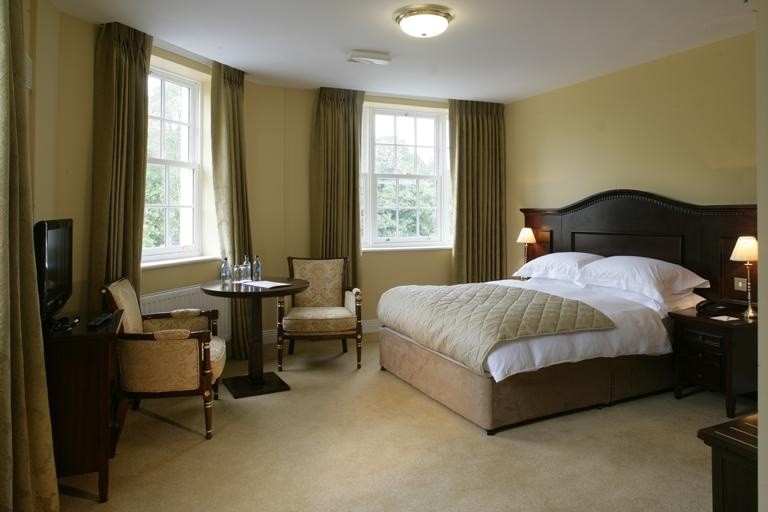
[43, 310, 130, 503]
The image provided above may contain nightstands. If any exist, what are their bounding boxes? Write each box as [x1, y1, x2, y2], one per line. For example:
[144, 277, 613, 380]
[665, 302, 758, 417]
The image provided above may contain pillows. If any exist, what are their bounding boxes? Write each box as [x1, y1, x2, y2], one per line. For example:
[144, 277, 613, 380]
[513, 250, 604, 284]
[575, 254, 712, 305]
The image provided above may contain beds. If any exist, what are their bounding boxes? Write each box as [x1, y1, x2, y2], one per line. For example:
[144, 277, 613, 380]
[375, 188, 759, 436]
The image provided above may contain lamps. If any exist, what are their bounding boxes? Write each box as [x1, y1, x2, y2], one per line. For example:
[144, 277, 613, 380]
[729, 235, 758, 318]
[393, 3, 454, 39]
[516, 227, 536, 263]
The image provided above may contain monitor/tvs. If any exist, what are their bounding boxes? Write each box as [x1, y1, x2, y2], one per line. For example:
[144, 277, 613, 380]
[33, 218, 74, 334]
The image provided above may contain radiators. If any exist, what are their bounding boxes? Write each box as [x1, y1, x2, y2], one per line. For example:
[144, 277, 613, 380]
[137, 280, 233, 353]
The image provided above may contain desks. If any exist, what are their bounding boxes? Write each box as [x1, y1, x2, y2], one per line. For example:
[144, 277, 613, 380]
[697, 414, 760, 509]
[205, 276, 310, 399]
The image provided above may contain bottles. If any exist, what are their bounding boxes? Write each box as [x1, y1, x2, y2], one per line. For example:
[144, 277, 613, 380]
[253, 255, 261, 282]
[243, 255, 252, 281]
[221, 257, 229, 281]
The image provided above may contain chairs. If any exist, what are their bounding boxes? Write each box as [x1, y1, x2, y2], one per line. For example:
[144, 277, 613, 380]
[276, 256, 363, 372]
[101, 274, 226, 439]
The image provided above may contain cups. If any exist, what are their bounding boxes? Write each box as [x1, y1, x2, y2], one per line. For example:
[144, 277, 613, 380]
[240, 267, 247, 279]
[233, 265, 240, 281]
